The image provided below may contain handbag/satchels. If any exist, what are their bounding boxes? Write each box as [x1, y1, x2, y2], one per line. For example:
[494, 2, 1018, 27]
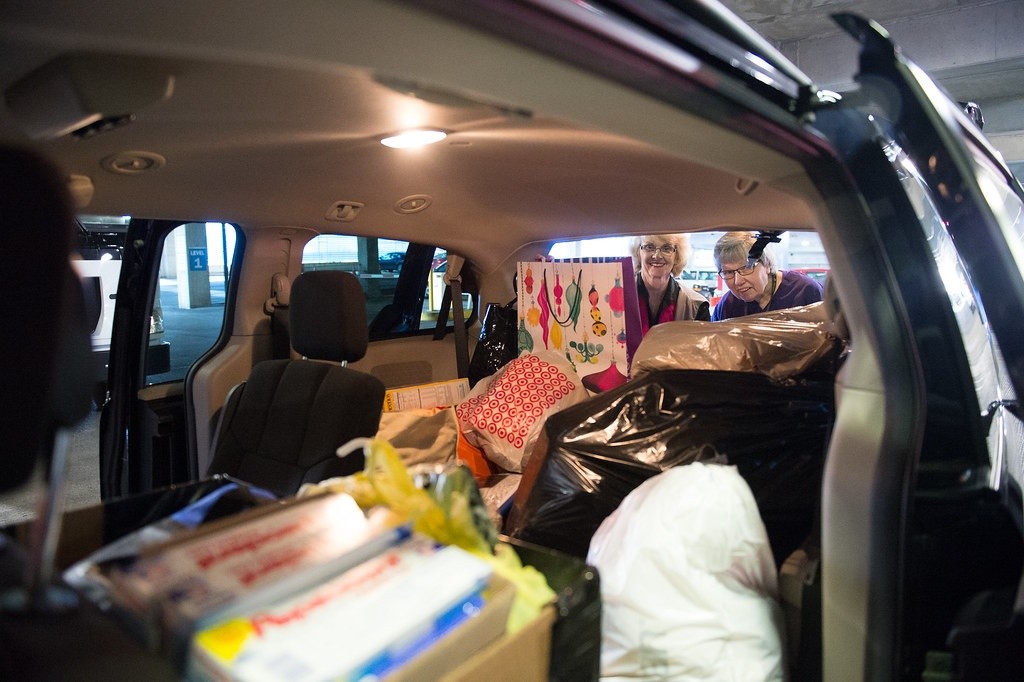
[374, 256, 642, 488]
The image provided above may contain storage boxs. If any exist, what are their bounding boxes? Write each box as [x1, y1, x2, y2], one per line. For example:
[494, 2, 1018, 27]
[45, 473, 602, 681]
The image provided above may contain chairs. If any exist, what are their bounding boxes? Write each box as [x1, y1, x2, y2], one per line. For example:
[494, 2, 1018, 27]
[187, 270, 387, 503]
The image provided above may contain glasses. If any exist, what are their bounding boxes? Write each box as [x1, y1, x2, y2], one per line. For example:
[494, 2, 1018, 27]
[718, 260, 760, 280]
[640, 242, 677, 253]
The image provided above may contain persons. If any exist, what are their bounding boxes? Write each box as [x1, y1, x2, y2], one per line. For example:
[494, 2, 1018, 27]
[709, 232, 824, 321]
[627, 233, 710, 341]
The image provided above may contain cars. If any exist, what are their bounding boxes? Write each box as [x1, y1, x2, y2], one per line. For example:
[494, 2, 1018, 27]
[423, 253, 450, 297]
[70, 215, 172, 381]
[379, 251, 405, 273]
